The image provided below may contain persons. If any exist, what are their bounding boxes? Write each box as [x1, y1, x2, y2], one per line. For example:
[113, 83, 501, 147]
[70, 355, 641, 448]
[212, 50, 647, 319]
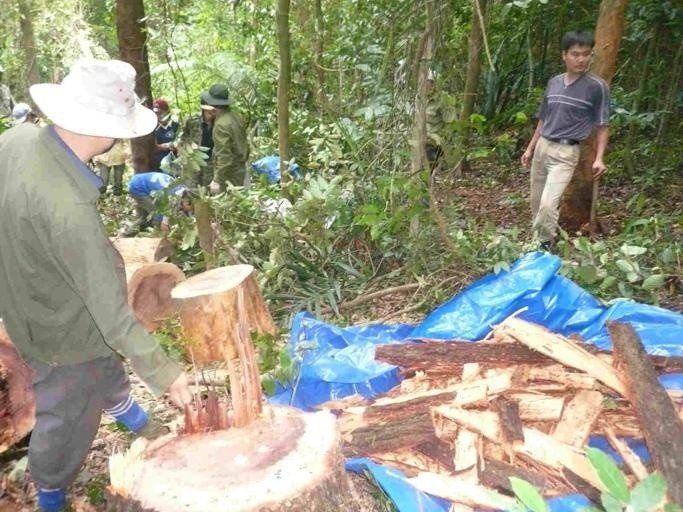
[12, 102, 37, 125]
[521, 28, 609, 250]
[152, 99, 182, 168]
[130, 172, 196, 232]
[423, 68, 454, 162]
[182, 92, 216, 186]
[0, 59, 194, 511]
[202, 85, 248, 196]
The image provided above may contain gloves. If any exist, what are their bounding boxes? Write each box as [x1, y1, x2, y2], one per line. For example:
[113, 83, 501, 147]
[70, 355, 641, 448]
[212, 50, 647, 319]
[208, 180, 220, 196]
[169, 373, 191, 409]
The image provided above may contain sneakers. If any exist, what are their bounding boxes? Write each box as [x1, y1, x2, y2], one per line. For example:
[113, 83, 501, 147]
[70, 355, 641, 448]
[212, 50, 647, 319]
[136, 420, 170, 440]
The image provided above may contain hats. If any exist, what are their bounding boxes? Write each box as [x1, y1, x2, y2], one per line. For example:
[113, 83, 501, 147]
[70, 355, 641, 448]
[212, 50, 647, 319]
[200, 99, 214, 110]
[12, 103, 32, 124]
[202, 85, 235, 105]
[153, 100, 169, 112]
[29, 59, 158, 139]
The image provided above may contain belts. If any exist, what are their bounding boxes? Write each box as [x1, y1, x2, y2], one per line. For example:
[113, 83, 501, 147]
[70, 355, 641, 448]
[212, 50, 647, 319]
[548, 138, 580, 145]
